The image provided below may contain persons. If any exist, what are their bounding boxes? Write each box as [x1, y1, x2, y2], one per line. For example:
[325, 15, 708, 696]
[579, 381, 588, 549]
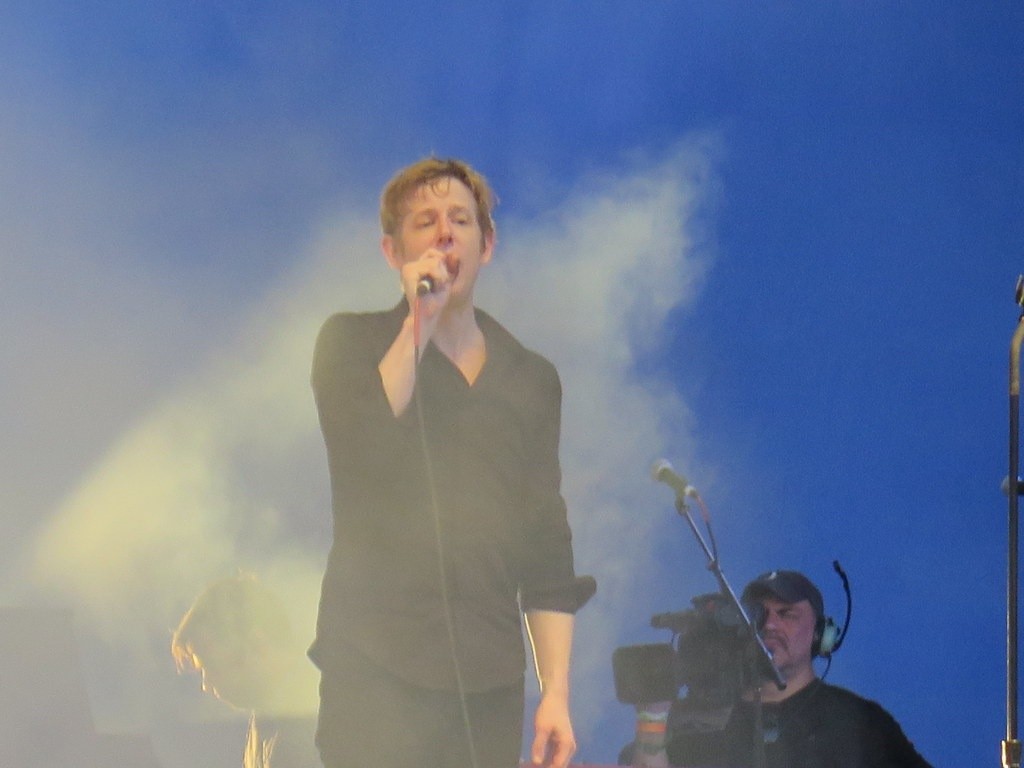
[172, 583, 325, 768]
[306, 150, 597, 768]
[618, 569, 930, 768]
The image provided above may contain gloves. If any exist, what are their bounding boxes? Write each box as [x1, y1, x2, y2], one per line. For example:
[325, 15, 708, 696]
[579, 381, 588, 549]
[635, 700, 672, 733]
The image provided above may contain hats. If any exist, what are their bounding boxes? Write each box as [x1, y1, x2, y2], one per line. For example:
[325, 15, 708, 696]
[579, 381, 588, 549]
[741, 571, 824, 619]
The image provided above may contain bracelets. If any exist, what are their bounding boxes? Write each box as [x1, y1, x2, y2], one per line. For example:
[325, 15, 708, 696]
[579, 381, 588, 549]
[637, 708, 668, 733]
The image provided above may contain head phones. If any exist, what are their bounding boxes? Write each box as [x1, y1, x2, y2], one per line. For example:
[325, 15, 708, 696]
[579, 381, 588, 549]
[740, 574, 841, 660]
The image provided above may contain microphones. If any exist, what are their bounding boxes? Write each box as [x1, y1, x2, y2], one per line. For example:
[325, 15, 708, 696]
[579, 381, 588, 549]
[651, 611, 696, 631]
[651, 457, 699, 498]
[417, 275, 435, 295]
[830, 560, 852, 654]
